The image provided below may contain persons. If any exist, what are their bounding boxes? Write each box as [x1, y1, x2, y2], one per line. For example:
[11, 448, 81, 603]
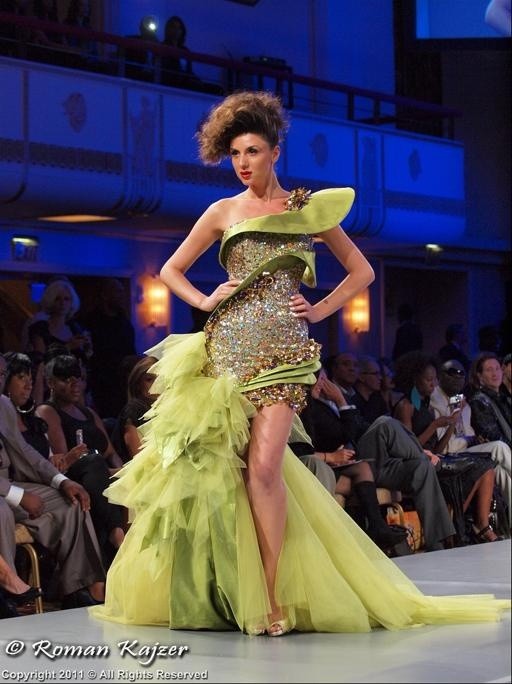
[1, 270, 511, 612]
[97, 89, 509, 635]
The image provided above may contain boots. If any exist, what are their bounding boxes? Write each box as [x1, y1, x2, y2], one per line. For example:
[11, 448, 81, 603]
[340, 479, 409, 552]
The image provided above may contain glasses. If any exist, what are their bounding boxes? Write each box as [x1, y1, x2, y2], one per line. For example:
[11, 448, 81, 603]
[360, 370, 380, 378]
[442, 368, 466, 378]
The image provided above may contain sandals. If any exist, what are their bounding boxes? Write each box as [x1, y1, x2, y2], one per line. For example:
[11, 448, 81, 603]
[249, 617, 289, 637]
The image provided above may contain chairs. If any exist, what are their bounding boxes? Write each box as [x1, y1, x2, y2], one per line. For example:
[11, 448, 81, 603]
[375, 488, 406, 531]
[14, 524, 45, 614]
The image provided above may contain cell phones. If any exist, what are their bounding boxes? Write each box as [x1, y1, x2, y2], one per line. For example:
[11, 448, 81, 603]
[450, 408, 460, 417]
[343, 439, 358, 451]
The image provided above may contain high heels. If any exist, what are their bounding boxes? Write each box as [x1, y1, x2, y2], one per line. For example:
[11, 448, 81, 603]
[473, 524, 504, 542]
[0, 576, 42, 607]
